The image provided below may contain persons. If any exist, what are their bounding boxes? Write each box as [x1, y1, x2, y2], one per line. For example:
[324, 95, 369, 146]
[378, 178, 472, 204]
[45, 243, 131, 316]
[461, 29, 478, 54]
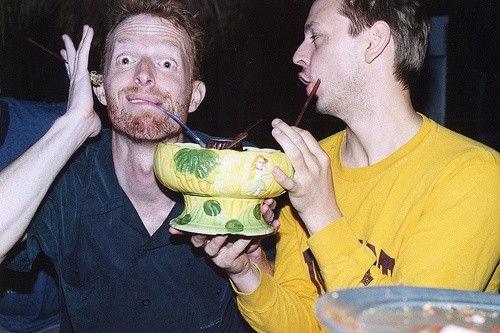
[0, 1, 281, 333]
[168, 0, 500, 333]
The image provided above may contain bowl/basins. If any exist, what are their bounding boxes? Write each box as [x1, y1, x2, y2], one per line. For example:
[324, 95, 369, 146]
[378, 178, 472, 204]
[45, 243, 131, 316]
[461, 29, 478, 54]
[153, 142, 294, 236]
[314, 286, 500, 333]
